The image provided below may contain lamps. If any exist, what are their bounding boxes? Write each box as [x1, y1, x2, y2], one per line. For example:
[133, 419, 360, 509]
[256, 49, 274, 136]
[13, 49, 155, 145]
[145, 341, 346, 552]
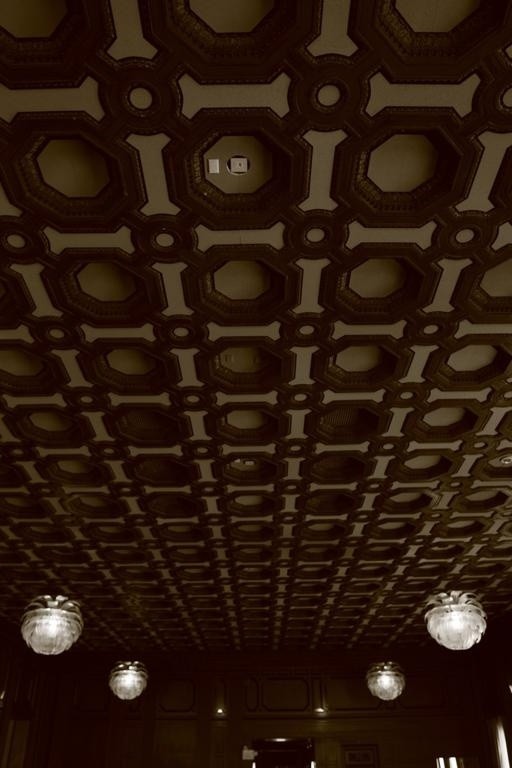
[106, 659, 148, 700]
[421, 591, 489, 651]
[363, 660, 409, 703]
[17, 593, 86, 656]
